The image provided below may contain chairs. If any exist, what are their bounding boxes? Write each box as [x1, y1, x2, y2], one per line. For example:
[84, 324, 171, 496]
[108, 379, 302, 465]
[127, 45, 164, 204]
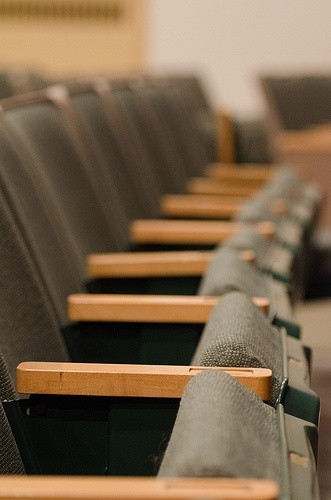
[0, 65, 331, 499]
